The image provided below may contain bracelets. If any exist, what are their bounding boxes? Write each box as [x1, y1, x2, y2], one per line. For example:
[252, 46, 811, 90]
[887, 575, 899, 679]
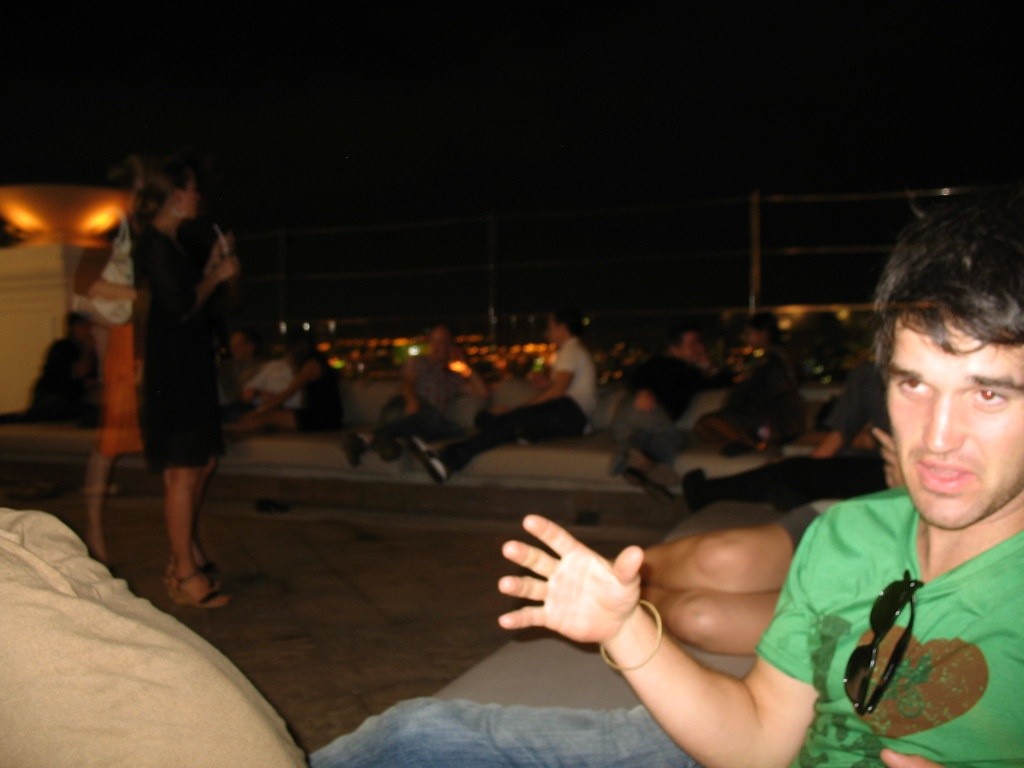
[600, 599, 662, 670]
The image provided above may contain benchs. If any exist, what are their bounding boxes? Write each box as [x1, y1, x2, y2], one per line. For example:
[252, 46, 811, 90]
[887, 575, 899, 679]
[1, 377, 817, 493]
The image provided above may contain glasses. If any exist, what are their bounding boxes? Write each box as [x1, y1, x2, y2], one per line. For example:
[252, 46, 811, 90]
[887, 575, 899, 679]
[844, 568, 924, 716]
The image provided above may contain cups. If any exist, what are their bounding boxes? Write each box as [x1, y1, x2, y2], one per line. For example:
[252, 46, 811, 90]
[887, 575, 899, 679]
[219, 246, 236, 275]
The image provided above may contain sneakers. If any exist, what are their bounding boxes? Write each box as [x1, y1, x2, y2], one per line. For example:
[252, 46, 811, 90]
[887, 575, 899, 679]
[411, 437, 449, 482]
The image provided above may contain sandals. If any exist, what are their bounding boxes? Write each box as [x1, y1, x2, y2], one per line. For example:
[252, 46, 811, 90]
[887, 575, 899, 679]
[165, 558, 231, 609]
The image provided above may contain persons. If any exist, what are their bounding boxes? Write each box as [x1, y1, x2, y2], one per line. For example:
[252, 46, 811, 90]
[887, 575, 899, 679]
[0, 505, 306, 768]
[306, 217, 1024, 768]
[216, 311, 602, 485]
[609, 316, 910, 656]
[63, 152, 242, 611]
